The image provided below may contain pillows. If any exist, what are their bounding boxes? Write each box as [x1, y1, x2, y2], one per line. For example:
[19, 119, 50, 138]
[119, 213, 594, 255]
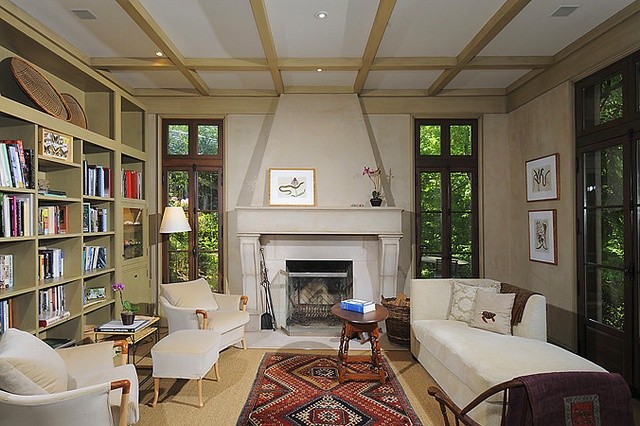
[447, 280, 496, 324]
[1, 327, 70, 396]
[469, 290, 517, 336]
[159, 278, 218, 311]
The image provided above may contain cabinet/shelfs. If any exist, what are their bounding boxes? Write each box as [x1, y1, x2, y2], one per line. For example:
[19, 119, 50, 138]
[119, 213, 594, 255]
[121, 88, 149, 318]
[95, 315, 160, 390]
[0, 6, 120, 349]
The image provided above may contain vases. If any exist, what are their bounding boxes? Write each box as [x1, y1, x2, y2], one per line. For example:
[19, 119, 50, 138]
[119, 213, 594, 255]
[370, 197, 382, 207]
[120, 312, 135, 325]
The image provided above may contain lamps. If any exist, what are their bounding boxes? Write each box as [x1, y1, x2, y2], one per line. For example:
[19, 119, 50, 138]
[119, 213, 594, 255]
[158, 206, 192, 282]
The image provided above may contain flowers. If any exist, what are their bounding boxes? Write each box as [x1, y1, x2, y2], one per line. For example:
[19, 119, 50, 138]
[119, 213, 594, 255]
[363, 163, 383, 198]
[110, 281, 139, 312]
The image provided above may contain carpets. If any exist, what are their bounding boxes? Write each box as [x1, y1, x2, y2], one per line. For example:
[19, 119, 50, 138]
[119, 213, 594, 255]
[235, 351, 424, 426]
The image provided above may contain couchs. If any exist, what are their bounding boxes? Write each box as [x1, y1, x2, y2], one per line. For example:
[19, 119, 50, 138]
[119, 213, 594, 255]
[156, 293, 248, 352]
[0, 327, 140, 426]
[408, 276, 610, 426]
[151, 330, 222, 409]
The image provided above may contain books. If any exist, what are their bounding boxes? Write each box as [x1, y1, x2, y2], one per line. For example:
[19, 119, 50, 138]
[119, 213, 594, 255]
[83, 203, 108, 234]
[0, 254, 14, 291]
[121, 167, 143, 200]
[0, 193, 34, 237]
[0, 299, 13, 336]
[83, 160, 114, 198]
[38, 246, 63, 281]
[340, 299, 376, 314]
[0, 140, 35, 189]
[94, 319, 150, 333]
[38, 204, 68, 235]
[83, 242, 107, 273]
[39, 285, 70, 327]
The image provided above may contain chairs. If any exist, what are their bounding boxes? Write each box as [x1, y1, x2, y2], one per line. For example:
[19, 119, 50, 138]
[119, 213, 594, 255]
[428, 372, 632, 425]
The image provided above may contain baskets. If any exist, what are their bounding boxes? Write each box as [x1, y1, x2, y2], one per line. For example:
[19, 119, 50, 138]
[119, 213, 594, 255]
[382, 296, 410, 348]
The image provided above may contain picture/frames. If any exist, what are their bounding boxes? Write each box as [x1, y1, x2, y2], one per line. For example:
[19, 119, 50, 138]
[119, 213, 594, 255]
[267, 167, 318, 207]
[525, 152, 561, 202]
[527, 209, 559, 266]
